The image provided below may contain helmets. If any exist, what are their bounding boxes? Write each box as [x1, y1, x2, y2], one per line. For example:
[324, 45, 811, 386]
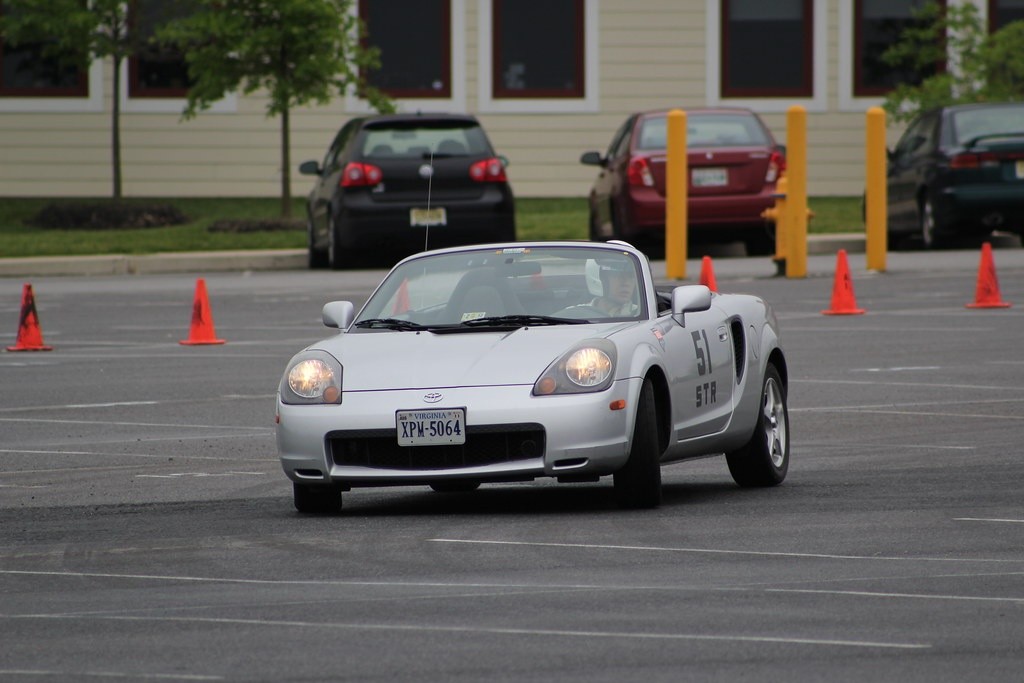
[585, 239, 636, 297]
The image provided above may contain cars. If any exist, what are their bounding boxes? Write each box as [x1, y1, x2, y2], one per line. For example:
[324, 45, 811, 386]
[579, 106, 784, 257]
[299, 112, 514, 273]
[863, 101, 1024, 252]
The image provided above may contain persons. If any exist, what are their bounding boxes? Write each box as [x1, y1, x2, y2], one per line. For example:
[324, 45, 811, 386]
[563, 240, 643, 315]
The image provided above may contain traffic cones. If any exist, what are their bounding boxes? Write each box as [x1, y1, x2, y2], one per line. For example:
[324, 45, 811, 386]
[701, 254, 716, 290]
[964, 242, 1010, 308]
[395, 280, 412, 313]
[5, 283, 56, 351]
[181, 278, 229, 344]
[821, 250, 863, 313]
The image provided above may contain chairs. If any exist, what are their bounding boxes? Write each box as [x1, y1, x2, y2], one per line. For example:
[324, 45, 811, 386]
[442, 269, 527, 322]
[438, 141, 466, 153]
[371, 145, 392, 154]
[565, 256, 639, 305]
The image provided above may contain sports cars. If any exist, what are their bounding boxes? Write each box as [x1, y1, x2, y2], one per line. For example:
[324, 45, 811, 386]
[275, 240, 790, 516]
